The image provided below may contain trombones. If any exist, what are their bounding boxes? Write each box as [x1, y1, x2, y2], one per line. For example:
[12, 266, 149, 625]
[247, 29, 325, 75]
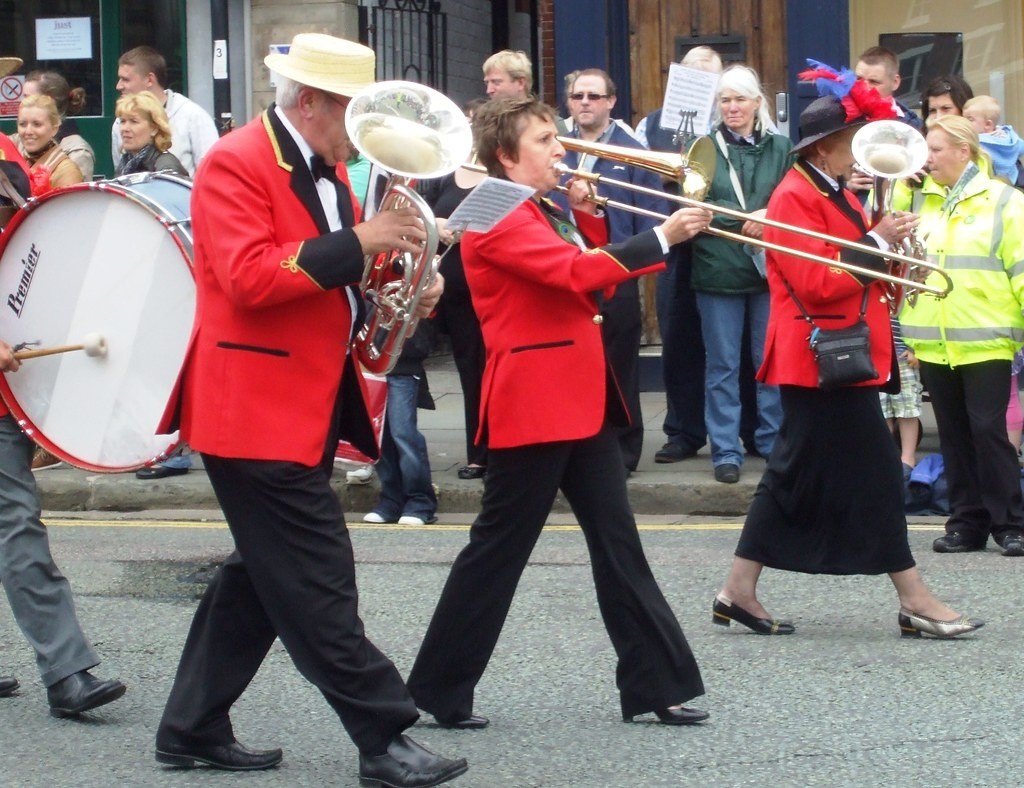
[454, 127, 956, 304]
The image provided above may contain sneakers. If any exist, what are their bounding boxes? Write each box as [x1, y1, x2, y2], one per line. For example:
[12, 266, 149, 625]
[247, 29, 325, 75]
[933, 532, 987, 553]
[31, 448, 63, 473]
[656, 443, 697, 462]
[346, 465, 373, 484]
[1001, 535, 1024, 557]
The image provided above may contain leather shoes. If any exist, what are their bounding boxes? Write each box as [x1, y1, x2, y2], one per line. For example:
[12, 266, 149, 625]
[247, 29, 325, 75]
[0, 676, 19, 696]
[715, 463, 739, 482]
[47, 671, 126, 716]
[155, 737, 283, 771]
[360, 735, 469, 788]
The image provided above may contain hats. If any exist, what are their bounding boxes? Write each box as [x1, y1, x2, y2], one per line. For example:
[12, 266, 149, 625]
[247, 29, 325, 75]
[787, 94, 865, 154]
[0, 57, 23, 80]
[263, 33, 376, 98]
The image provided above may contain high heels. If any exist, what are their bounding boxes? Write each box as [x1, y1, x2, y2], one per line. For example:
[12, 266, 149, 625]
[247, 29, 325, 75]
[434, 714, 489, 729]
[623, 708, 709, 725]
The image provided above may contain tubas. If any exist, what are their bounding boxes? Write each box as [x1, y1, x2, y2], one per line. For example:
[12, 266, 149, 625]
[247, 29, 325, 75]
[851, 118, 932, 317]
[342, 76, 477, 376]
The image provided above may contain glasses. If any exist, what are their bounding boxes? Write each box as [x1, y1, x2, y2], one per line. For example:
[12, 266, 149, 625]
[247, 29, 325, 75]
[571, 94, 611, 100]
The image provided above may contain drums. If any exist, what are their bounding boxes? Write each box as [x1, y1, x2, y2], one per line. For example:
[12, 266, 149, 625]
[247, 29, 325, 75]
[0, 168, 199, 473]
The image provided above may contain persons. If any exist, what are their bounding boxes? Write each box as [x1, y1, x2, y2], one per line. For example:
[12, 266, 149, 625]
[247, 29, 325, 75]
[153, 33, 469, 788]
[0, 131, 126, 717]
[405, 87, 714, 732]
[712, 97, 987, 638]
[0, 47, 1024, 557]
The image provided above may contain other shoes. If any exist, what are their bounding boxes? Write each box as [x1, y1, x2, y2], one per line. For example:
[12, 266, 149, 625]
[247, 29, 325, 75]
[397, 516, 425, 526]
[898, 607, 985, 638]
[713, 597, 795, 636]
[458, 466, 487, 478]
[364, 512, 387, 523]
[902, 463, 914, 480]
[136, 466, 187, 479]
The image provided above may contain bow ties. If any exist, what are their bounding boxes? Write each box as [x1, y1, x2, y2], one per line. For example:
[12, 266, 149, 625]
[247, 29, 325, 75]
[310, 155, 335, 183]
[838, 174, 849, 192]
